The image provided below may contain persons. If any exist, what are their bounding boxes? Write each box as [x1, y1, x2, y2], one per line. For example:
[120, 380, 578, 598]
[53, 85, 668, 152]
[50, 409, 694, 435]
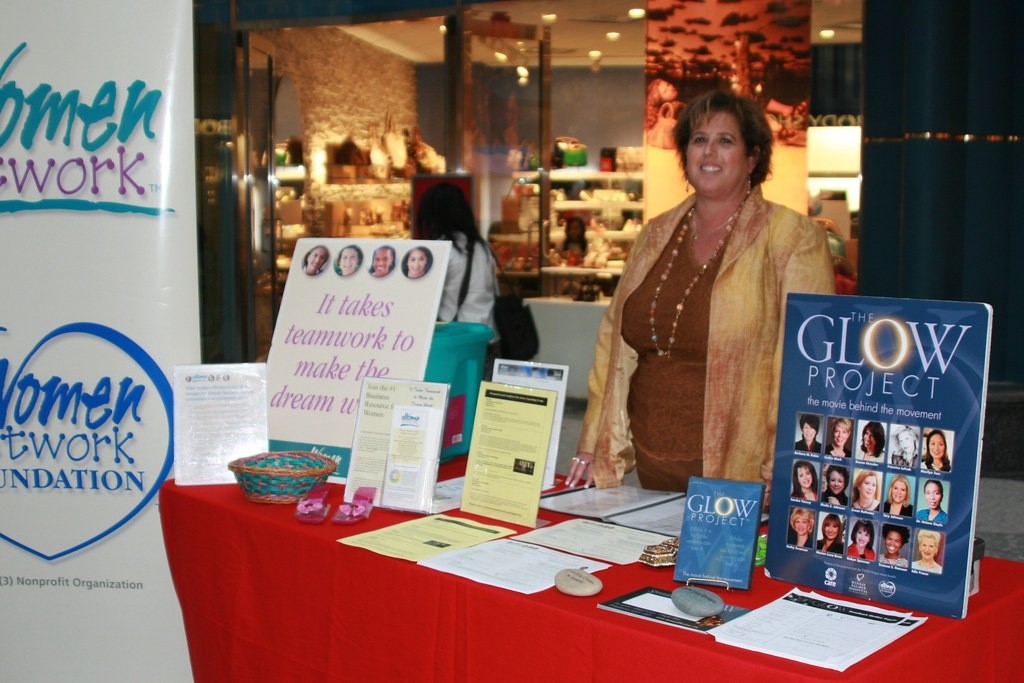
[280, 134, 307, 198]
[414, 183, 500, 382]
[562, 215, 588, 258]
[785, 411, 956, 576]
[299, 245, 433, 280]
[565, 90, 836, 520]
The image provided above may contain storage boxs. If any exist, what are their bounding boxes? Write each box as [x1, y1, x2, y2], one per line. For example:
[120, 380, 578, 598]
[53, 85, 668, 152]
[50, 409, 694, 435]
[422, 321, 496, 464]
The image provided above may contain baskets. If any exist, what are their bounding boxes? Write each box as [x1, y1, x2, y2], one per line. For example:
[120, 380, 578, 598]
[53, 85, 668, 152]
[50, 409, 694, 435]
[227, 450, 337, 504]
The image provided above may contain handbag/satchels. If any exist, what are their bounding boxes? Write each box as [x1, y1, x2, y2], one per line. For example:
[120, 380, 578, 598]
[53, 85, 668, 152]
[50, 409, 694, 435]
[482, 252, 540, 369]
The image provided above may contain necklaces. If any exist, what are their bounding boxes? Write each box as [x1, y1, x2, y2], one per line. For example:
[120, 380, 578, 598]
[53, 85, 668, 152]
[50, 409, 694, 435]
[650, 197, 744, 361]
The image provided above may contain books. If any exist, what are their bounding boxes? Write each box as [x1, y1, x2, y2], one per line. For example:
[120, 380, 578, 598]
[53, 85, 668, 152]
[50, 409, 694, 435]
[672, 476, 767, 591]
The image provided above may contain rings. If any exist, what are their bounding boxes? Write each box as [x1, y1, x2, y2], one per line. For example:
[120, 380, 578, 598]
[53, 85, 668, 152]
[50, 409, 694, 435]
[571, 457, 580, 462]
[580, 460, 588, 465]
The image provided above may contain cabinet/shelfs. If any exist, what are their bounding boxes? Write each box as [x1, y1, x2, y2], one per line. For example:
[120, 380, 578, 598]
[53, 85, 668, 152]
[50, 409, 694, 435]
[275, 161, 646, 295]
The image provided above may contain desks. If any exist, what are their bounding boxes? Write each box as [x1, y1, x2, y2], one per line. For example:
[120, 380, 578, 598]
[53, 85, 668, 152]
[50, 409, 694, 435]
[524, 298, 613, 405]
[159, 456, 1023, 683]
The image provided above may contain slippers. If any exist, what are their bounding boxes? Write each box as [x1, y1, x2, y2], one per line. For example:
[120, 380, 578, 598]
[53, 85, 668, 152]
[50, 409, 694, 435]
[331, 486, 376, 523]
[297, 483, 333, 523]
